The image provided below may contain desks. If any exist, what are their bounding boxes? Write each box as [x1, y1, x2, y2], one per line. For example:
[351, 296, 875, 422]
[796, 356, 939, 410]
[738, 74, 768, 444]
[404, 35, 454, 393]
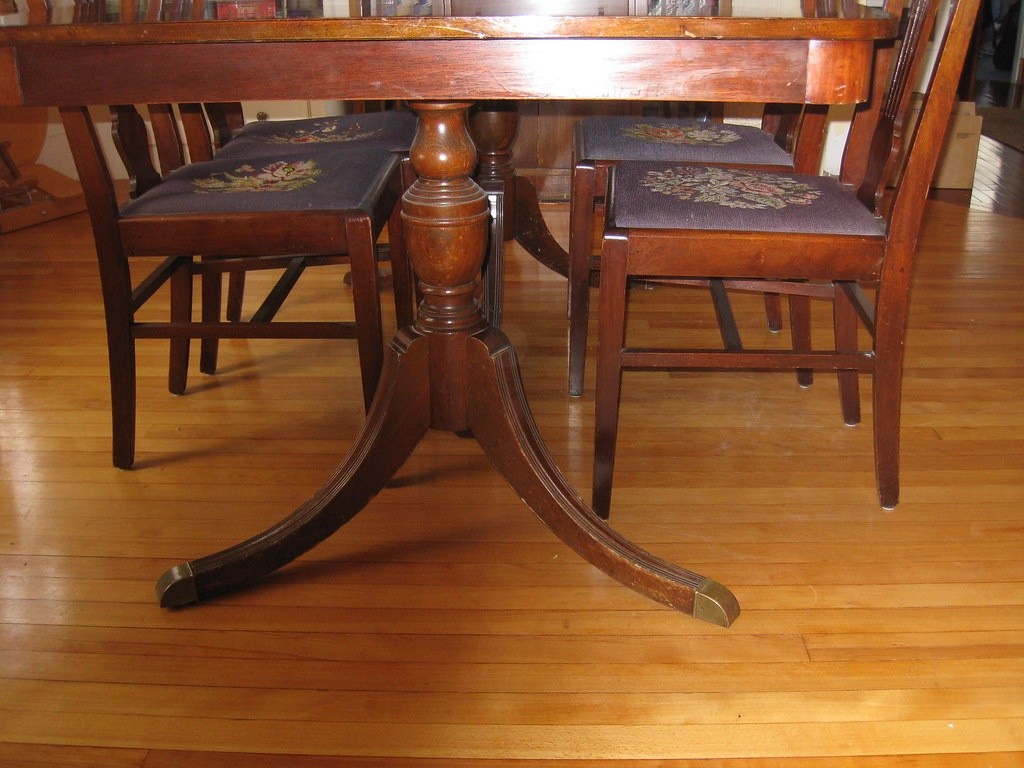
[0, 15, 897, 628]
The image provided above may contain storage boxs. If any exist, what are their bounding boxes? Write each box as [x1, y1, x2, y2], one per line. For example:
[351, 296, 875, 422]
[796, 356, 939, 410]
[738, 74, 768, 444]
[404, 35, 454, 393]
[0, 162, 87, 235]
[888, 91, 984, 190]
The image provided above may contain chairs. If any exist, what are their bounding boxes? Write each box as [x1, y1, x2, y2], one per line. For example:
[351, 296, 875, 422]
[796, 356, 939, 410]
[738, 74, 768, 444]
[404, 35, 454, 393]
[27, 0, 417, 471]
[593, 0, 979, 524]
[566, 0, 840, 399]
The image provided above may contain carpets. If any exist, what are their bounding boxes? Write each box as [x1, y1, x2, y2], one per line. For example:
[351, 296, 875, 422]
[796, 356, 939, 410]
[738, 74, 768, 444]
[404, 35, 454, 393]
[975, 107, 1024, 151]
